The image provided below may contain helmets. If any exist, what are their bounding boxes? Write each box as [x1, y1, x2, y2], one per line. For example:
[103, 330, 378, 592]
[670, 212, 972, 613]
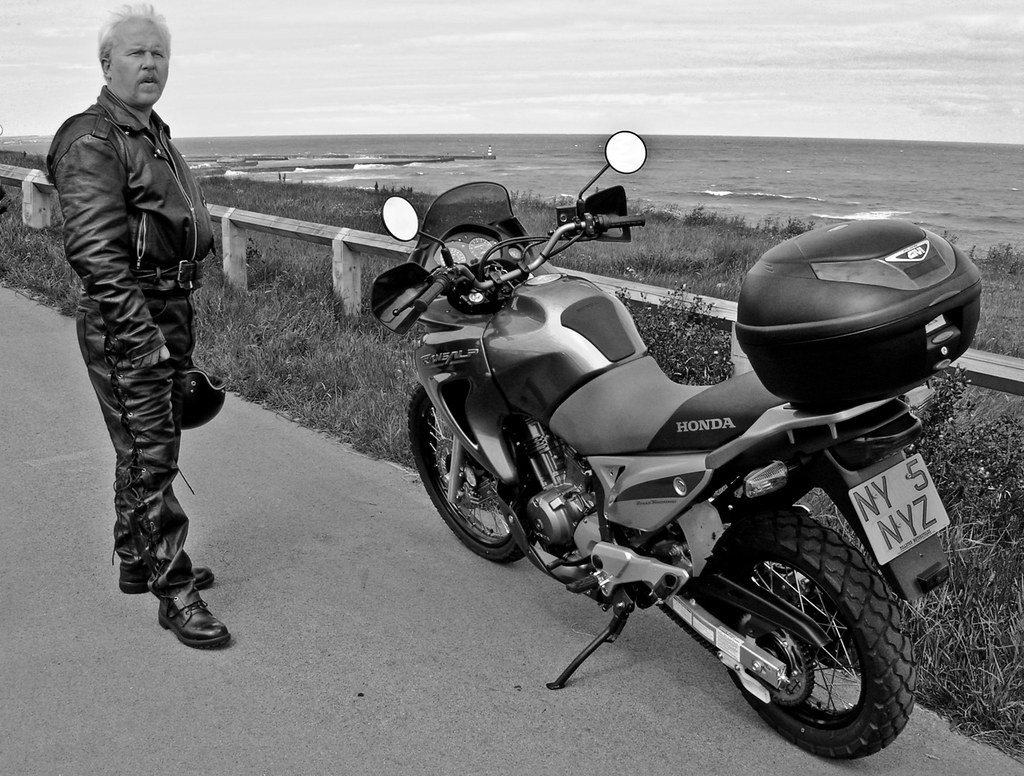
[172, 368, 226, 430]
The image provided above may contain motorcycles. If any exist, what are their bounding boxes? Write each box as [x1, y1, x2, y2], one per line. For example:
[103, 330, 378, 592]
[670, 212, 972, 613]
[371, 130, 985, 760]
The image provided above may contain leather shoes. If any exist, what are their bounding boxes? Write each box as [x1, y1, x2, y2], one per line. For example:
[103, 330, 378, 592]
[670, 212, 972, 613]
[159, 598, 230, 648]
[120, 559, 215, 595]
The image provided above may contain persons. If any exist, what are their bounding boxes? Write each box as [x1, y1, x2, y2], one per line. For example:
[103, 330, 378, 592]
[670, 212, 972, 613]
[45, 4, 232, 648]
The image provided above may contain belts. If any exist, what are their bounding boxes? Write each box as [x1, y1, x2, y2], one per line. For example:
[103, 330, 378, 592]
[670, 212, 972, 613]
[128, 256, 207, 283]
